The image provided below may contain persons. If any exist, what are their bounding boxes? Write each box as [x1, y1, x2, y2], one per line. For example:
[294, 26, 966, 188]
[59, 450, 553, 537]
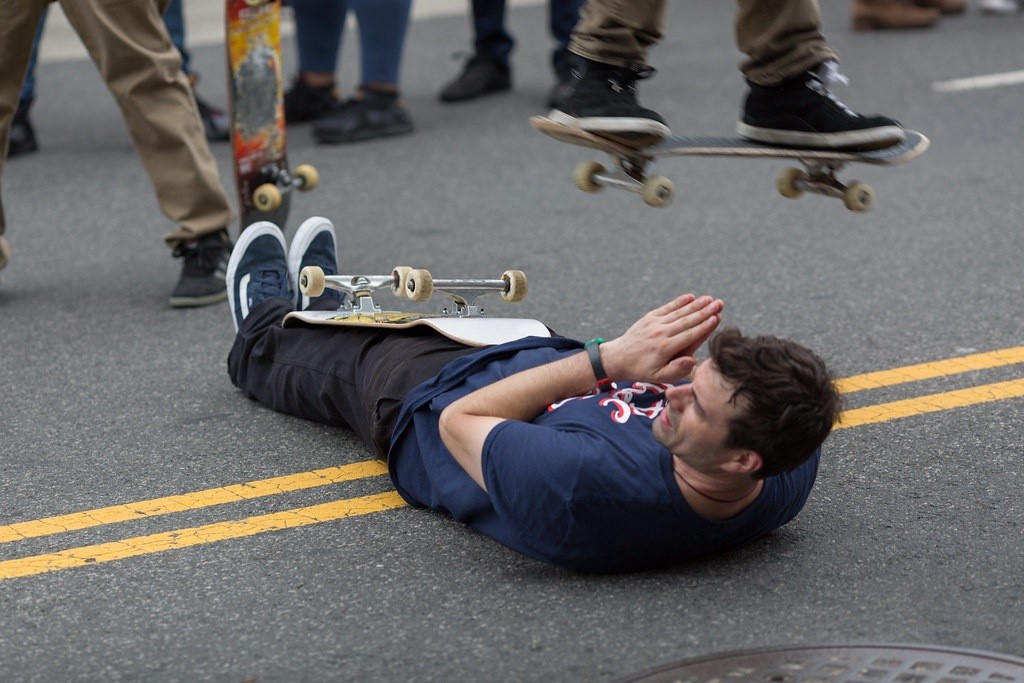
[852, 0, 964, 33]
[225, 216, 845, 569]
[280, 1, 418, 142]
[435, 0, 583, 103]
[0, 0, 236, 307]
[7, 1, 228, 156]
[548, 0, 905, 152]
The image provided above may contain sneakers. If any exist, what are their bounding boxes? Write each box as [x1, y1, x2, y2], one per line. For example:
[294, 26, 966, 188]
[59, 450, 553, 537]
[549, 49, 672, 149]
[438, 56, 509, 101]
[738, 57, 907, 153]
[288, 216, 344, 311]
[312, 87, 414, 144]
[169, 229, 233, 306]
[225, 222, 288, 333]
[286, 77, 338, 122]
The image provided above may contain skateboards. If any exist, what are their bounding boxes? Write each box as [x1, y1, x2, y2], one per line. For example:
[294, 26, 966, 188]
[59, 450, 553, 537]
[222, 1, 317, 233]
[527, 114, 932, 212]
[280, 263, 552, 348]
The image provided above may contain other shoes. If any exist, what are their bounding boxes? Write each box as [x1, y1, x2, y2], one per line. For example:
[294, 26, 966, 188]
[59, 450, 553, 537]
[196, 95, 231, 141]
[852, 2, 940, 31]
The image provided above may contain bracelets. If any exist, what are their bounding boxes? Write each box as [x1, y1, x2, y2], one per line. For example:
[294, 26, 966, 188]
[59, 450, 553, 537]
[584, 338, 613, 392]
